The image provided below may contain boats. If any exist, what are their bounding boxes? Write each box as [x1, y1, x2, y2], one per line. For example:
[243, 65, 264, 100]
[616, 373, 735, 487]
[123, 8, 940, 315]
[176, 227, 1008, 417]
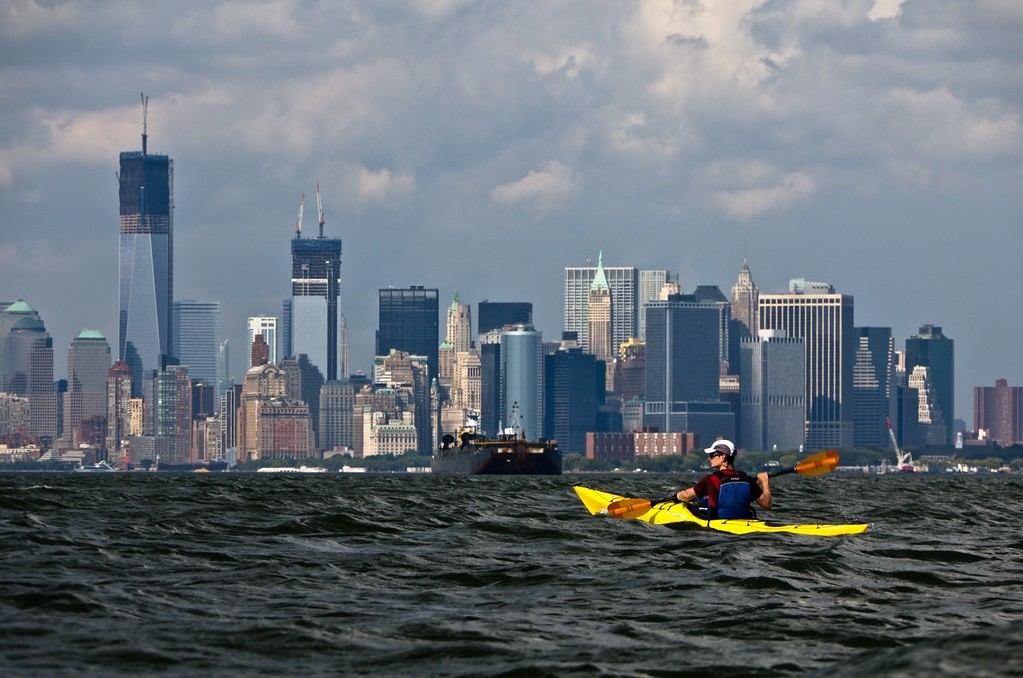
[572, 481, 871, 539]
[427, 402, 548, 475]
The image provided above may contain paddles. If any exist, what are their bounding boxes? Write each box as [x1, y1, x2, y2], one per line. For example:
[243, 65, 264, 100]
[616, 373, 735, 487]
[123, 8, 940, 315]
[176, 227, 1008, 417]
[608, 451, 840, 521]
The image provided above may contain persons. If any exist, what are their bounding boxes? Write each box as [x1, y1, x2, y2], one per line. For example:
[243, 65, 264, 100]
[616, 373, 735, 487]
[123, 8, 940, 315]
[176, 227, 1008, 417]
[677, 440, 771, 520]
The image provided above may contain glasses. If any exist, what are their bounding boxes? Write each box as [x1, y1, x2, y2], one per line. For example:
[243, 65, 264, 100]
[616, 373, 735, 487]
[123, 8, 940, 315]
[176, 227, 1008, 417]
[710, 454, 721, 459]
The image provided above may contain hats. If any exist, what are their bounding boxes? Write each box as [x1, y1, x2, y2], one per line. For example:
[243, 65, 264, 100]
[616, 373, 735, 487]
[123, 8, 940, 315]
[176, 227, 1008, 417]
[704, 440, 736, 457]
